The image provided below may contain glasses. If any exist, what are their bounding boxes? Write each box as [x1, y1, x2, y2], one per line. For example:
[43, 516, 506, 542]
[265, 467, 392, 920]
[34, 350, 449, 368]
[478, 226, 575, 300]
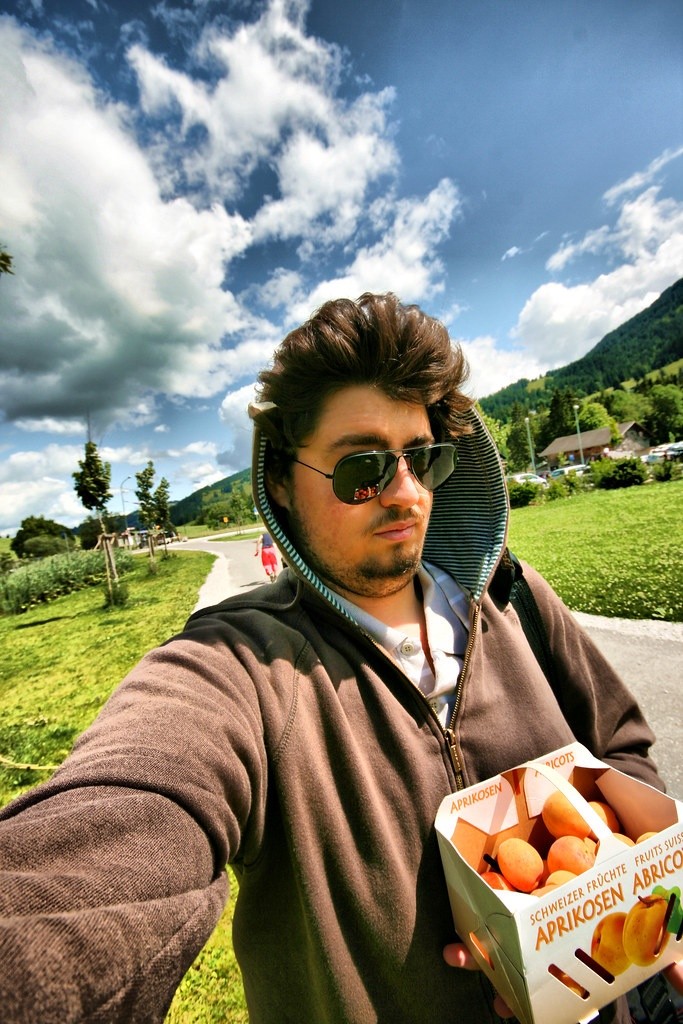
[292, 442, 460, 504]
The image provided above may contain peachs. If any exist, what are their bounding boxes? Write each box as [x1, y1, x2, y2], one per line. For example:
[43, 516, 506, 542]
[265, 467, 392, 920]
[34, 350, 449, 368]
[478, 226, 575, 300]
[589, 894, 673, 976]
[474, 790, 660, 995]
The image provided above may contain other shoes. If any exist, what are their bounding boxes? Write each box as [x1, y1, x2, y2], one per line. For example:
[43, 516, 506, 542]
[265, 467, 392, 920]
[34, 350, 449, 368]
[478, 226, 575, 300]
[270, 574, 277, 583]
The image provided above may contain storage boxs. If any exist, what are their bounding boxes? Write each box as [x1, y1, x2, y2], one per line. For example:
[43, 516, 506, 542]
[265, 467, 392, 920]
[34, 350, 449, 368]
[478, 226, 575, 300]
[431, 740, 683, 1024]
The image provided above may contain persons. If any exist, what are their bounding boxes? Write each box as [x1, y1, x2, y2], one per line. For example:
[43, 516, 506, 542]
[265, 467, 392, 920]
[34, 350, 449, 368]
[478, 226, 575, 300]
[0, 291, 683, 1024]
[254, 532, 278, 582]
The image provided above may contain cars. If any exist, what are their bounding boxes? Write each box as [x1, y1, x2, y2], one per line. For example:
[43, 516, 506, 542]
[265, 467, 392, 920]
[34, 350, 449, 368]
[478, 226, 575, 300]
[551, 463, 590, 480]
[505, 473, 547, 488]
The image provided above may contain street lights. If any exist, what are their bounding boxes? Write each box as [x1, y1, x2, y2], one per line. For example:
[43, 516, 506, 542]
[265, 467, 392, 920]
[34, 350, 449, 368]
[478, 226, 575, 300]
[120, 476, 133, 531]
[524, 417, 536, 475]
[572, 404, 586, 465]
